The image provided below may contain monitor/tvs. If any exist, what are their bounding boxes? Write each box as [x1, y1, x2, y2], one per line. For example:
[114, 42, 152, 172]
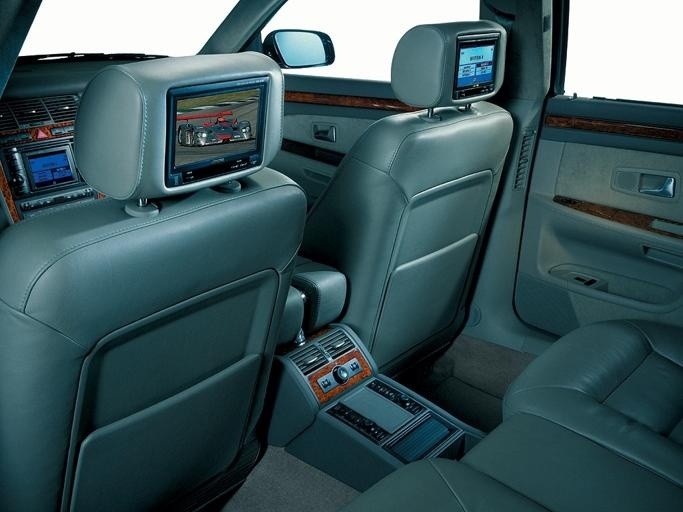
[166, 73, 269, 185]
[453, 33, 501, 99]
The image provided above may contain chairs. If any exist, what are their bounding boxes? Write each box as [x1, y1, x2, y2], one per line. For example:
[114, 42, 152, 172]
[2, 45, 301, 509]
[341, 318, 681, 507]
[307, 12, 524, 374]
